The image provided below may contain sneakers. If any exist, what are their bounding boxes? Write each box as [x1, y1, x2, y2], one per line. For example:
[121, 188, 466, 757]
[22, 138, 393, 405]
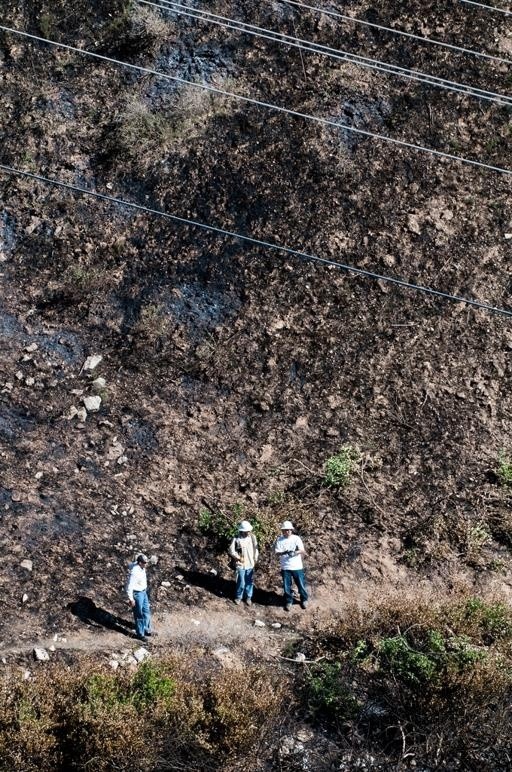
[301, 601, 310, 608]
[234, 598, 242, 604]
[244, 600, 253, 606]
[138, 630, 157, 643]
[285, 602, 292, 611]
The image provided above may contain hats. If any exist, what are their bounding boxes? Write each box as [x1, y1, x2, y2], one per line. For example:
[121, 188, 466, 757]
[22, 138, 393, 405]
[135, 553, 150, 567]
[278, 520, 295, 532]
[237, 521, 254, 533]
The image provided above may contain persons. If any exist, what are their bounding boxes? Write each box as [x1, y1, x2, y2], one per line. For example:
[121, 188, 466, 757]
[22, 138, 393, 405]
[230, 521, 259, 605]
[274, 520, 309, 611]
[126, 555, 158, 642]
